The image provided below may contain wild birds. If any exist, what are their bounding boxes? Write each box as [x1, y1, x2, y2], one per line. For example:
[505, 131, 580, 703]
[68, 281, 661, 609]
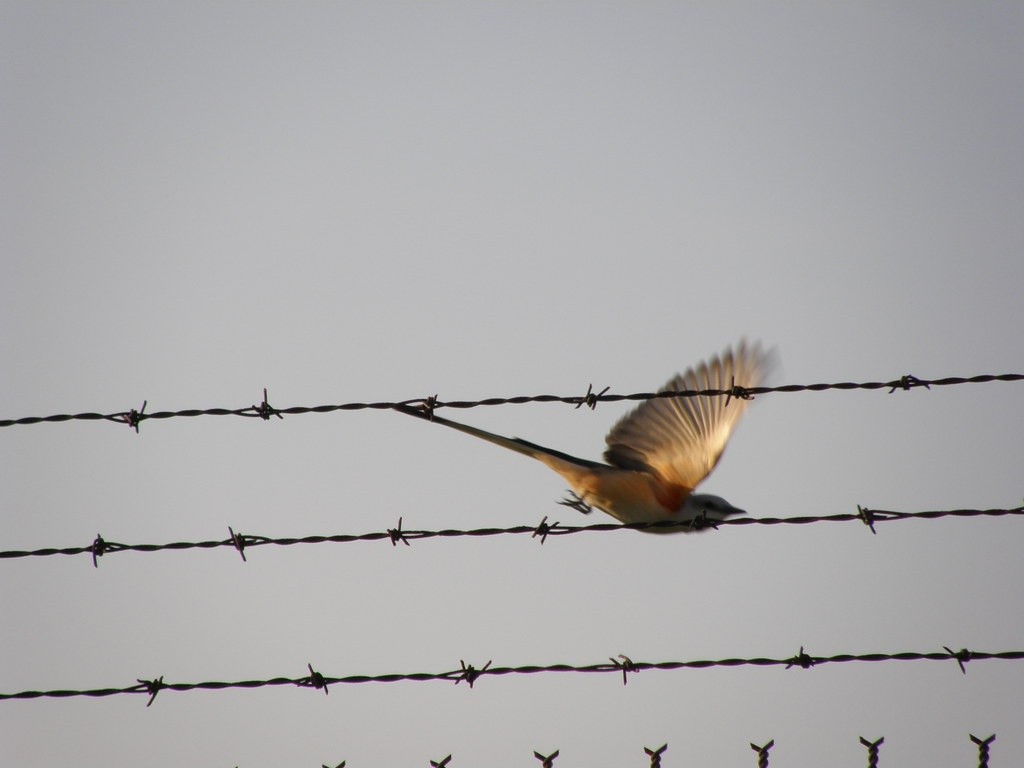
[399, 336, 775, 534]
